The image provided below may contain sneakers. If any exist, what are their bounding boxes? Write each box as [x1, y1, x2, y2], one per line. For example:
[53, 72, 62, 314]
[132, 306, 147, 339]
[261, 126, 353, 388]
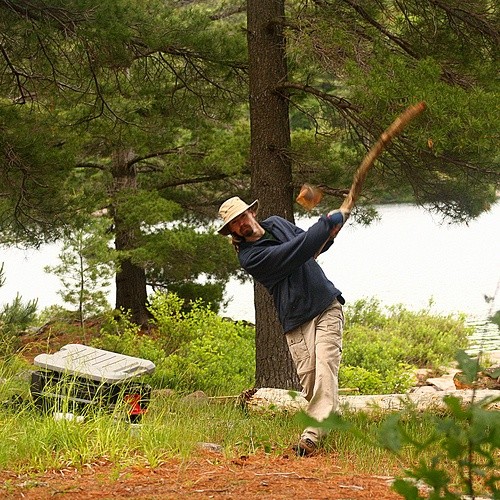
[293, 439, 323, 456]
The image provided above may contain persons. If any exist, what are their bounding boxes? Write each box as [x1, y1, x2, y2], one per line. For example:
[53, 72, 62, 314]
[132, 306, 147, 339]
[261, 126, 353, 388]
[216, 196, 352, 456]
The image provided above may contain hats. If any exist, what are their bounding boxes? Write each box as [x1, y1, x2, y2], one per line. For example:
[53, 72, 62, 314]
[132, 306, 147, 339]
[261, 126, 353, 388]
[216, 197, 260, 236]
